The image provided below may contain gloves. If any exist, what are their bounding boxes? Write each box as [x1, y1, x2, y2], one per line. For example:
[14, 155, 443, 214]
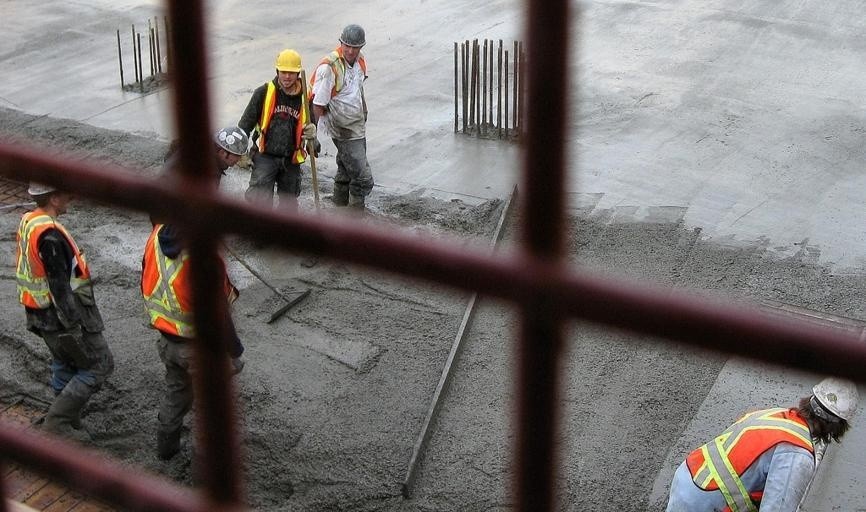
[304, 137, 320, 159]
[68, 324, 83, 344]
[232, 356, 245, 376]
[301, 123, 317, 139]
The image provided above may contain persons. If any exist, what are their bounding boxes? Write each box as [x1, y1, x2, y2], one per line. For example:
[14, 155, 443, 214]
[237, 49, 303, 218]
[303, 24, 374, 214]
[661, 376, 859, 512]
[138, 197, 244, 462]
[156, 128, 247, 191]
[15, 179, 114, 443]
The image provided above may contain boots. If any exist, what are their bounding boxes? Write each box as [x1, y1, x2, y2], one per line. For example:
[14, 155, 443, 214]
[334, 184, 350, 206]
[157, 412, 187, 461]
[349, 193, 366, 210]
[41, 393, 91, 444]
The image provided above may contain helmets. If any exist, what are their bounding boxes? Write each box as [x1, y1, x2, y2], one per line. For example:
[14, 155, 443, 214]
[812, 376, 859, 422]
[215, 126, 250, 155]
[340, 25, 366, 47]
[275, 49, 302, 72]
[27, 181, 55, 196]
